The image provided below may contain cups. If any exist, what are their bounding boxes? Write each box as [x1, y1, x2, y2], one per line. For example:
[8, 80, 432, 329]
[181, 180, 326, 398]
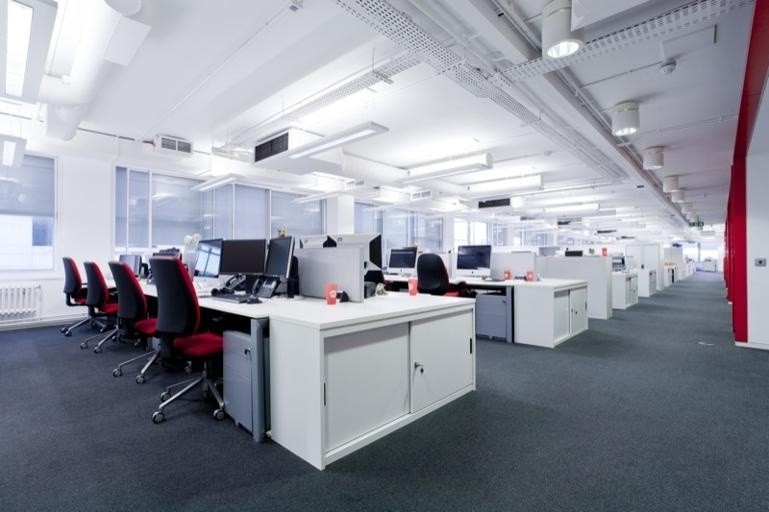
[325, 283, 337, 304]
[375, 282, 385, 295]
[602, 248, 607, 256]
[504, 270, 511, 279]
[527, 271, 533, 280]
[408, 277, 418, 296]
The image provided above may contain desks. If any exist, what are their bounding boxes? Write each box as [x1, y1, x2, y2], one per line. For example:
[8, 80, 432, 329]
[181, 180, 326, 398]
[381, 270, 588, 349]
[104, 276, 477, 470]
[613, 264, 678, 311]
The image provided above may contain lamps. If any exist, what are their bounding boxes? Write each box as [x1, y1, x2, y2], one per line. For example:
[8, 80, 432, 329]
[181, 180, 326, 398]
[610, 103, 641, 137]
[539, 0, 585, 63]
[661, 176, 699, 224]
[642, 145, 665, 172]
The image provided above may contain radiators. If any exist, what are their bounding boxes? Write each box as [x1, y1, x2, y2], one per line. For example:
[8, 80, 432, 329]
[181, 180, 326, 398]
[0, 284, 42, 323]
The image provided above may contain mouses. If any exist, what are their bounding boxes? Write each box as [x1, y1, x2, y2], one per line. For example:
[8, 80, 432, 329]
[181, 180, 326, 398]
[485, 277, 493, 280]
[247, 297, 260, 303]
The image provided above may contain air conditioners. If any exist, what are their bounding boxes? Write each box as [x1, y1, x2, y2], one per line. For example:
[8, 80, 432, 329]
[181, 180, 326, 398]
[152, 135, 193, 158]
[477, 198, 523, 214]
[254, 128, 342, 178]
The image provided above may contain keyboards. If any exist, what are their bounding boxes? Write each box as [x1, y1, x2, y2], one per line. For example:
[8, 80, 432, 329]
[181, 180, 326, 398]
[213, 294, 251, 303]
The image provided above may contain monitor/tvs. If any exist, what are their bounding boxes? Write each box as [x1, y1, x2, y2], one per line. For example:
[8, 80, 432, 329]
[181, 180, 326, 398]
[387, 248, 418, 274]
[194, 238, 224, 279]
[323, 233, 382, 277]
[297, 234, 323, 249]
[264, 235, 295, 279]
[453, 243, 493, 280]
[119, 255, 142, 276]
[219, 239, 267, 276]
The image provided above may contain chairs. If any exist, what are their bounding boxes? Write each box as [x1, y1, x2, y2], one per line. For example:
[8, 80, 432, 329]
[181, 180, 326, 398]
[415, 253, 465, 297]
[53, 254, 226, 425]
